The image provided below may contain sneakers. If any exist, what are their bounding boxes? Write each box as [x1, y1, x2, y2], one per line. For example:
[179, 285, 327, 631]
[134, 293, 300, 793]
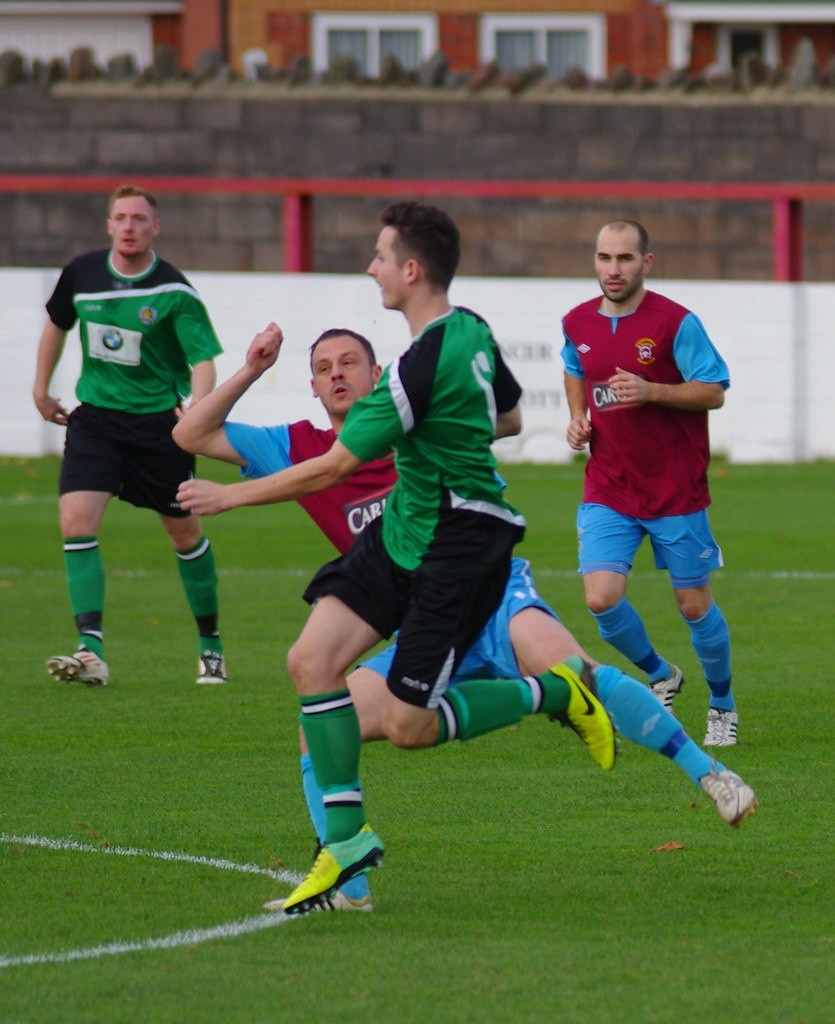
[197, 649, 230, 685]
[47, 645, 110, 685]
[263, 887, 376, 914]
[703, 708, 738, 747]
[700, 758, 759, 829]
[283, 820, 386, 914]
[548, 654, 623, 772]
[653, 662, 686, 721]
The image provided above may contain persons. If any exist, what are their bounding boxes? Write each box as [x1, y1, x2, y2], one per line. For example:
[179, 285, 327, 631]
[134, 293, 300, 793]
[561, 220, 737, 750]
[0, 47, 835, 95]
[173, 201, 758, 917]
[33, 186, 228, 686]
